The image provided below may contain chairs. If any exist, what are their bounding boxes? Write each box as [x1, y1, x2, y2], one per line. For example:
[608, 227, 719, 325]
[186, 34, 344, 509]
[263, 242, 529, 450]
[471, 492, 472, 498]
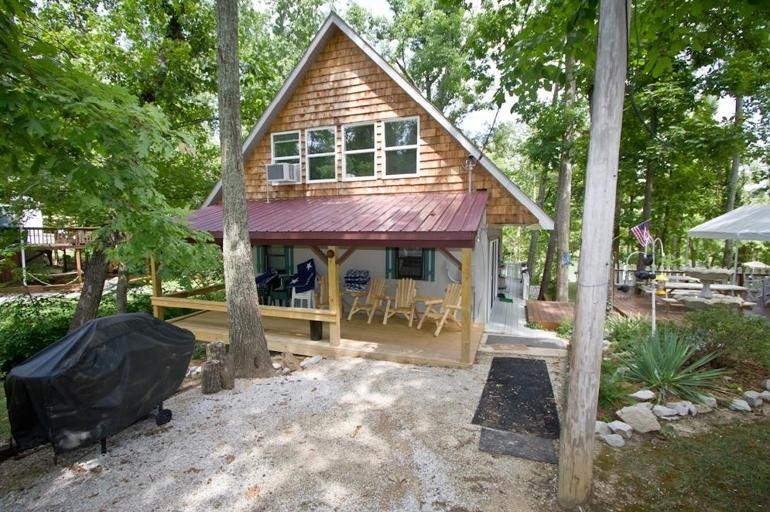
[346, 276, 386, 324]
[383, 277, 419, 328]
[270, 277, 290, 307]
[417, 279, 461, 337]
[291, 273, 320, 308]
[256, 271, 279, 305]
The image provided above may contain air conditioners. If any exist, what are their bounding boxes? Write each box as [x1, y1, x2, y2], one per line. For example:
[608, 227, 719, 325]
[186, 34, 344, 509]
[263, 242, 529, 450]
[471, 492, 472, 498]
[289, 162, 301, 180]
[266, 162, 289, 180]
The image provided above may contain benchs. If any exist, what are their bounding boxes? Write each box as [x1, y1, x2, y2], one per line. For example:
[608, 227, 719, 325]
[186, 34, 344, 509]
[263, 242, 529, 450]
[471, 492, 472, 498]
[636, 275, 757, 310]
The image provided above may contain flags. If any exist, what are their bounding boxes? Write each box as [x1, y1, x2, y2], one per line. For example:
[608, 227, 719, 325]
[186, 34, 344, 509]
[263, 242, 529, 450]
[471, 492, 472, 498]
[631, 220, 654, 248]
